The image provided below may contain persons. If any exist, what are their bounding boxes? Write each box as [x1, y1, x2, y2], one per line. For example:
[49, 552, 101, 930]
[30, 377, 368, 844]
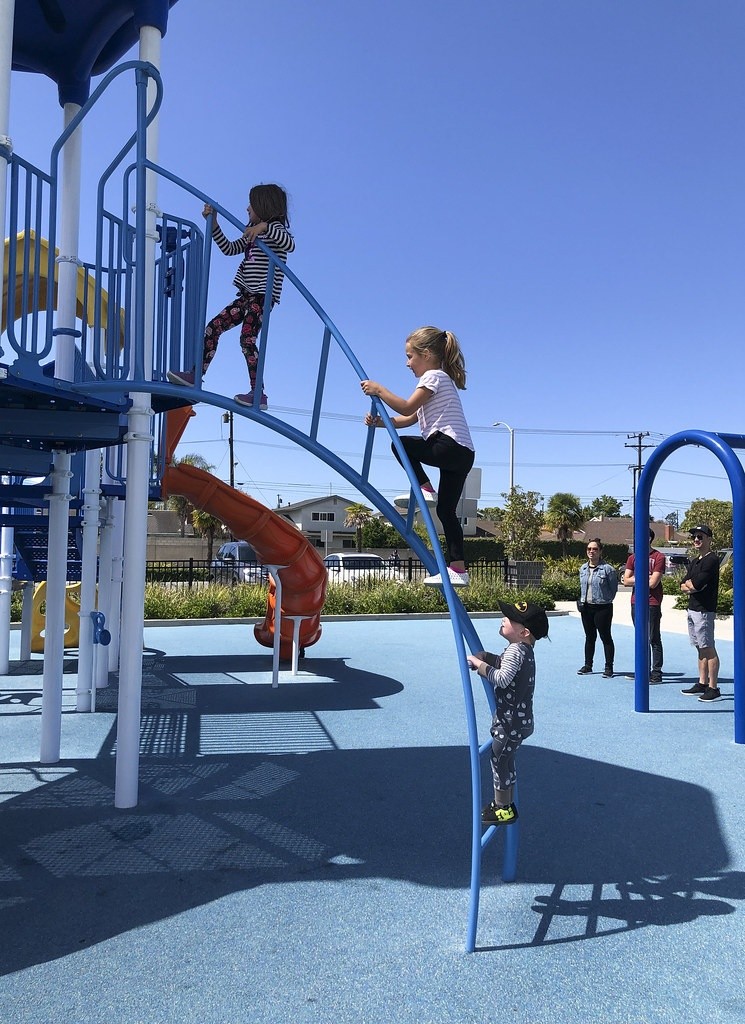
[577, 538, 619, 678]
[623, 528, 667, 685]
[165, 183, 296, 411]
[360, 325, 476, 588]
[679, 524, 724, 703]
[466, 599, 550, 824]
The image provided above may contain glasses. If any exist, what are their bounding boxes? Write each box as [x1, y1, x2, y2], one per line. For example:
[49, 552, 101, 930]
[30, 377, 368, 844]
[587, 546, 601, 552]
[691, 535, 703, 540]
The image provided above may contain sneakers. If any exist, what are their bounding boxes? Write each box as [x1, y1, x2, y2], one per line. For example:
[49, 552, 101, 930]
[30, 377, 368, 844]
[393, 488, 438, 509]
[649, 675, 662, 685]
[577, 666, 594, 675]
[680, 683, 709, 695]
[697, 687, 722, 702]
[625, 674, 635, 680]
[482, 800, 519, 826]
[234, 390, 268, 410]
[423, 565, 468, 588]
[602, 670, 613, 678]
[167, 370, 204, 387]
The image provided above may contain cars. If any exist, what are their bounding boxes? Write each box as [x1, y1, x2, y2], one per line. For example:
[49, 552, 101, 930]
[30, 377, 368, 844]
[617, 553, 688, 584]
[323, 553, 403, 586]
[210, 542, 270, 584]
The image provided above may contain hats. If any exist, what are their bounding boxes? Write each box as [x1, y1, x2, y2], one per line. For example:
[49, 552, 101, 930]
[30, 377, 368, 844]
[497, 600, 548, 640]
[689, 525, 713, 537]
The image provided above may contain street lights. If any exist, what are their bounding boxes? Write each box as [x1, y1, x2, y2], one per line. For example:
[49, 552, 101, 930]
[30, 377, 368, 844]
[493, 422, 515, 542]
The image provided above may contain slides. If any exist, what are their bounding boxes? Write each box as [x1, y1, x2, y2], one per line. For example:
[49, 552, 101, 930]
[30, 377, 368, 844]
[162, 461, 329, 648]
